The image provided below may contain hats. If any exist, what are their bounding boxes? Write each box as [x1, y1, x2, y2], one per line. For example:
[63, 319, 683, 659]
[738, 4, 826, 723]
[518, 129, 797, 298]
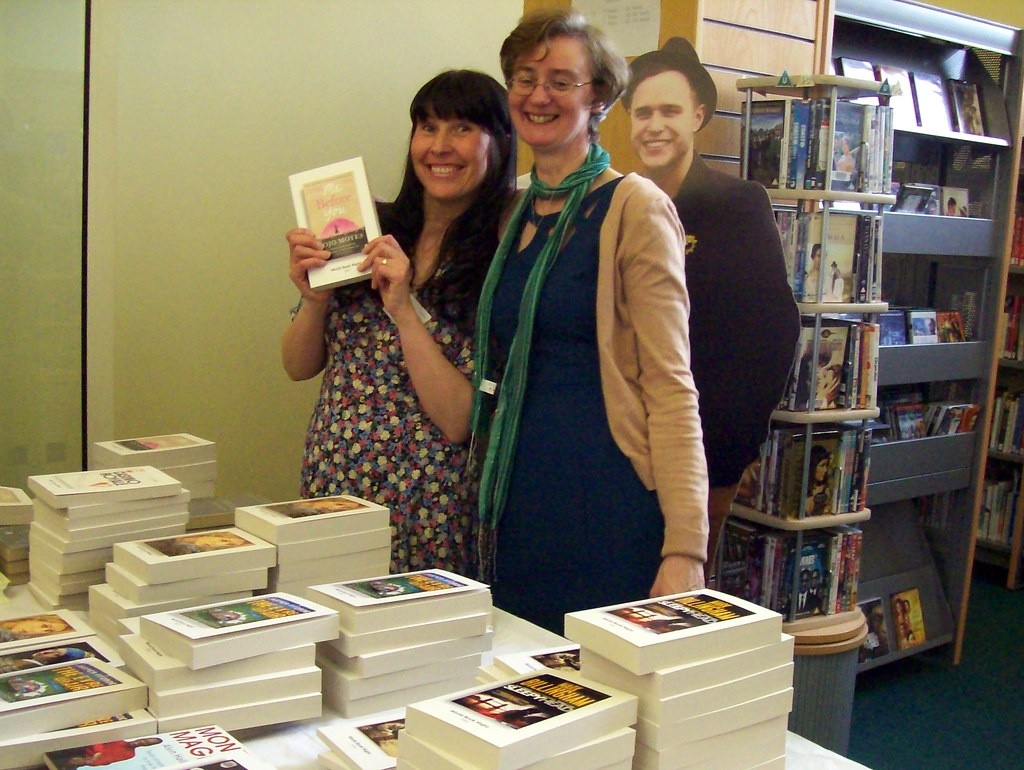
[620, 37, 717, 133]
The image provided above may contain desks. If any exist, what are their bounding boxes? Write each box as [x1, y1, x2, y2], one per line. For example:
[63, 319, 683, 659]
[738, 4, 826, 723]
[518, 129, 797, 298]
[224, 606, 872, 770]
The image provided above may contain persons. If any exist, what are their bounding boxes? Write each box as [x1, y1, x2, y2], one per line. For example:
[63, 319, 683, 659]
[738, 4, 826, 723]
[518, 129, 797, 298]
[0, 647, 85, 674]
[282, 69, 518, 584]
[810, 570, 819, 595]
[788, 569, 819, 614]
[837, 137, 854, 172]
[945, 197, 956, 216]
[146, 534, 254, 556]
[0, 616, 71, 641]
[266, 499, 362, 518]
[371, 9, 709, 637]
[832, 134, 840, 171]
[960, 206, 968, 217]
[808, 244, 833, 302]
[806, 445, 832, 514]
[621, 37, 800, 589]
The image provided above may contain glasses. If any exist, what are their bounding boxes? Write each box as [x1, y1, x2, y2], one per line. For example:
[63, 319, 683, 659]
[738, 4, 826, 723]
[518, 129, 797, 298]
[506, 77, 596, 97]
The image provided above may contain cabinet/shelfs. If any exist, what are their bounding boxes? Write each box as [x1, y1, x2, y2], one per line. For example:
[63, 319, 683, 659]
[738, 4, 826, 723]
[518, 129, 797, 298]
[715, 67, 897, 634]
[826, 0, 1024, 674]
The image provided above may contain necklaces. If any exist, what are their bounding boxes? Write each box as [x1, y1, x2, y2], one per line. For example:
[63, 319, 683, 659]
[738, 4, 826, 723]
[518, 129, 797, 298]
[531, 179, 560, 236]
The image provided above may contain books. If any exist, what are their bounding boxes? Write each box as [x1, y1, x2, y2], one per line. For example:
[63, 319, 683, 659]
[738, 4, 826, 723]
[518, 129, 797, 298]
[0, 434, 795, 769]
[715, 60, 1023, 666]
[288, 156, 383, 290]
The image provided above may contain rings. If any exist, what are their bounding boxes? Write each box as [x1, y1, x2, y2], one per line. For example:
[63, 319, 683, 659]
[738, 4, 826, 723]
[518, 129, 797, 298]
[383, 257, 388, 266]
